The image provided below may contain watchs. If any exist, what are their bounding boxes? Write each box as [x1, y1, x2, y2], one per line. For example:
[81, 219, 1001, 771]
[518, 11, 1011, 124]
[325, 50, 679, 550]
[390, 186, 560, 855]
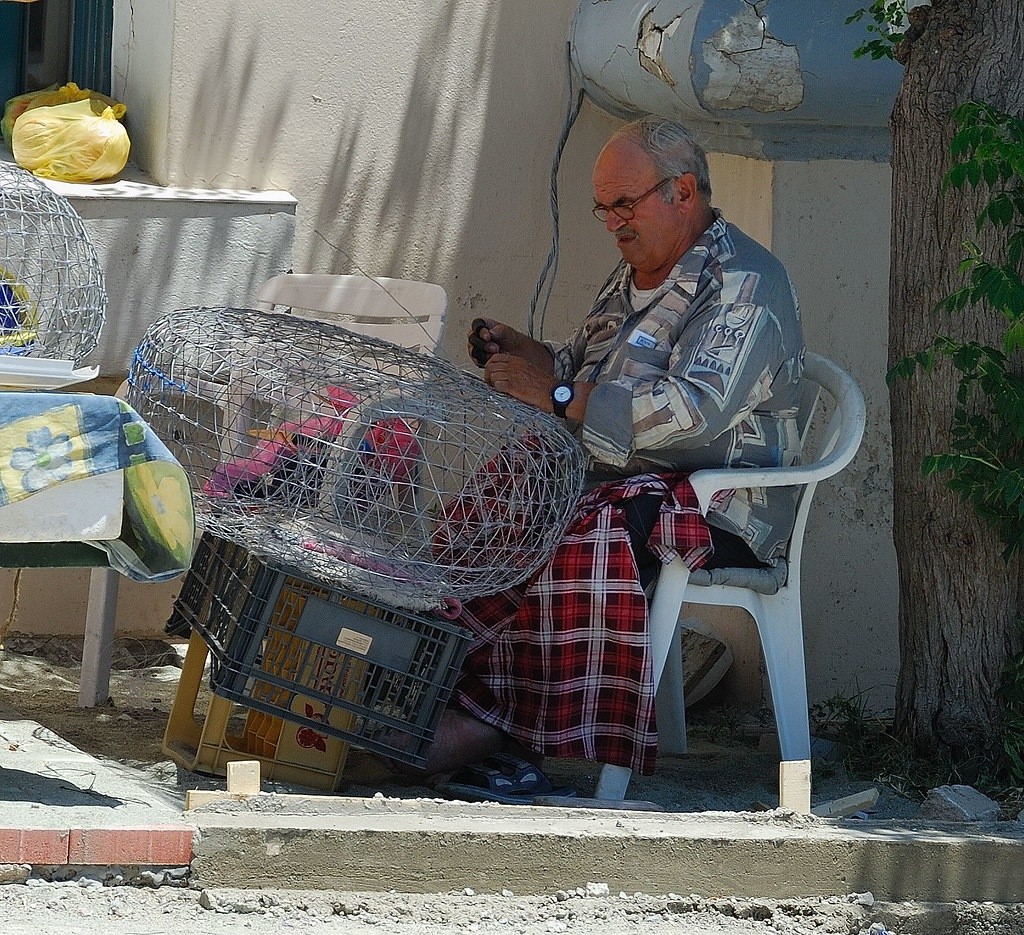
[551, 380, 574, 419]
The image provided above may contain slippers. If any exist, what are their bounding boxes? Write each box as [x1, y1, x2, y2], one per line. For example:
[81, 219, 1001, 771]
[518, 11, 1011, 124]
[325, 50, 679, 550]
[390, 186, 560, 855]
[434, 751, 577, 805]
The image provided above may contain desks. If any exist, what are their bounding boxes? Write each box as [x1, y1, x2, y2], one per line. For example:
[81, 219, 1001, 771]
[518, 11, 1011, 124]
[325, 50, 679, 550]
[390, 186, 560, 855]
[0, 391, 195, 708]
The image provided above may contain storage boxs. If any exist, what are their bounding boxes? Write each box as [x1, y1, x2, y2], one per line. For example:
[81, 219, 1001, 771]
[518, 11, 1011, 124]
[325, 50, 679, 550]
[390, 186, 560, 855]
[163, 530, 469, 794]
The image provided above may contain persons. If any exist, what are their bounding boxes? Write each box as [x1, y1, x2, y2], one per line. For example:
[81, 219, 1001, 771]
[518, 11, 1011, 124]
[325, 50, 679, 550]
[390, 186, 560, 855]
[386, 119, 804, 796]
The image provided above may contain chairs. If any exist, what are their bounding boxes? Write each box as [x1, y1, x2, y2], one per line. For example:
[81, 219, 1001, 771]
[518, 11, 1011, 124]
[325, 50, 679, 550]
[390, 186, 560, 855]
[561, 347, 860, 808]
[76, 273, 446, 710]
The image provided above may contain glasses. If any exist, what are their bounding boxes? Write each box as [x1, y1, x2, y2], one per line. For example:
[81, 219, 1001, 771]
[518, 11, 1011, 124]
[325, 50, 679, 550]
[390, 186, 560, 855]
[592, 171, 691, 222]
